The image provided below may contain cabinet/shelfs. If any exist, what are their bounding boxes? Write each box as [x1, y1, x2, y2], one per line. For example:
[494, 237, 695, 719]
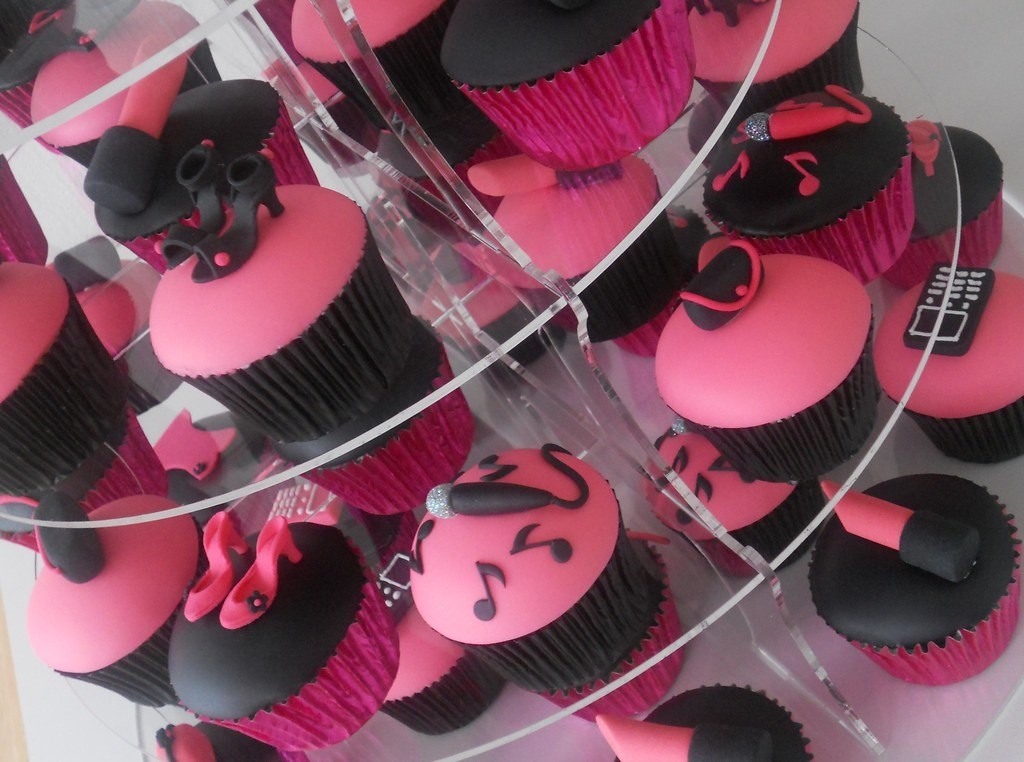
[0, 0, 1024, 762]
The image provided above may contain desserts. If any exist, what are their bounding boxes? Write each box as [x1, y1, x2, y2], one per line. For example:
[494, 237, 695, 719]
[0, 0, 1024, 762]
